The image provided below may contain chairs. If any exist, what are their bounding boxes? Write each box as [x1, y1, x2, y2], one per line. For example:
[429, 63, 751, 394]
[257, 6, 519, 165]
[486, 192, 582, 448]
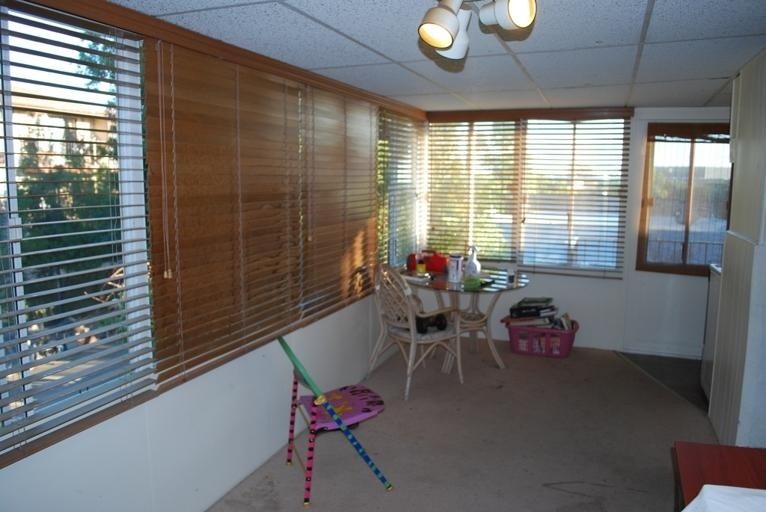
[368, 265, 465, 399]
[276, 336, 393, 508]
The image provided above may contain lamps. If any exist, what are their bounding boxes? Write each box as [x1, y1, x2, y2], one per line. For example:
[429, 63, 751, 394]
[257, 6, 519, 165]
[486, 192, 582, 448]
[417, 0, 536, 61]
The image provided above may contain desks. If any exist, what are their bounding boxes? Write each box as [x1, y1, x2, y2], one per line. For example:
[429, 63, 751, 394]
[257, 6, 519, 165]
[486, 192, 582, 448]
[671, 441, 766, 512]
[395, 269, 529, 371]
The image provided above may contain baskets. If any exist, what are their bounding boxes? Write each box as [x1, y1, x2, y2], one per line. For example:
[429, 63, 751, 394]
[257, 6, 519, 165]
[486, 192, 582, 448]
[505, 318, 579, 358]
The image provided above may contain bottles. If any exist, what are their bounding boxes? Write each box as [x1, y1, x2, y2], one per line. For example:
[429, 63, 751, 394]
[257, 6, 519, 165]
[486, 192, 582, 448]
[508, 266, 515, 286]
[448, 254, 464, 282]
[415, 248, 425, 276]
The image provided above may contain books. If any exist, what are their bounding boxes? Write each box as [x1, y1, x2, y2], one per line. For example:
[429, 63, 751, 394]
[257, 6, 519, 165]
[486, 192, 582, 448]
[499, 295, 574, 354]
